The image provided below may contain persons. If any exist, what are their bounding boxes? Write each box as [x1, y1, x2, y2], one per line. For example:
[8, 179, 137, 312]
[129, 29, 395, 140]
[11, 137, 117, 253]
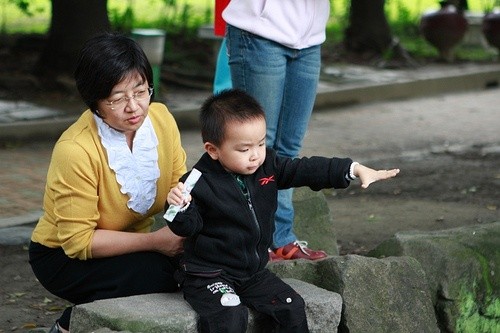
[164, 88, 401, 333]
[214, 0, 332, 263]
[28, 33, 193, 333]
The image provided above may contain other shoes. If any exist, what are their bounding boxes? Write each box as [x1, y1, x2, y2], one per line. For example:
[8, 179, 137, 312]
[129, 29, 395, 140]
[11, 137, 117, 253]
[268, 240, 328, 262]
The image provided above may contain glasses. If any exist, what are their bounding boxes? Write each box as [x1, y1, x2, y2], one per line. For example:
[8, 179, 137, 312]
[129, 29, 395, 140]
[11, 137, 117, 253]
[103, 87, 154, 110]
